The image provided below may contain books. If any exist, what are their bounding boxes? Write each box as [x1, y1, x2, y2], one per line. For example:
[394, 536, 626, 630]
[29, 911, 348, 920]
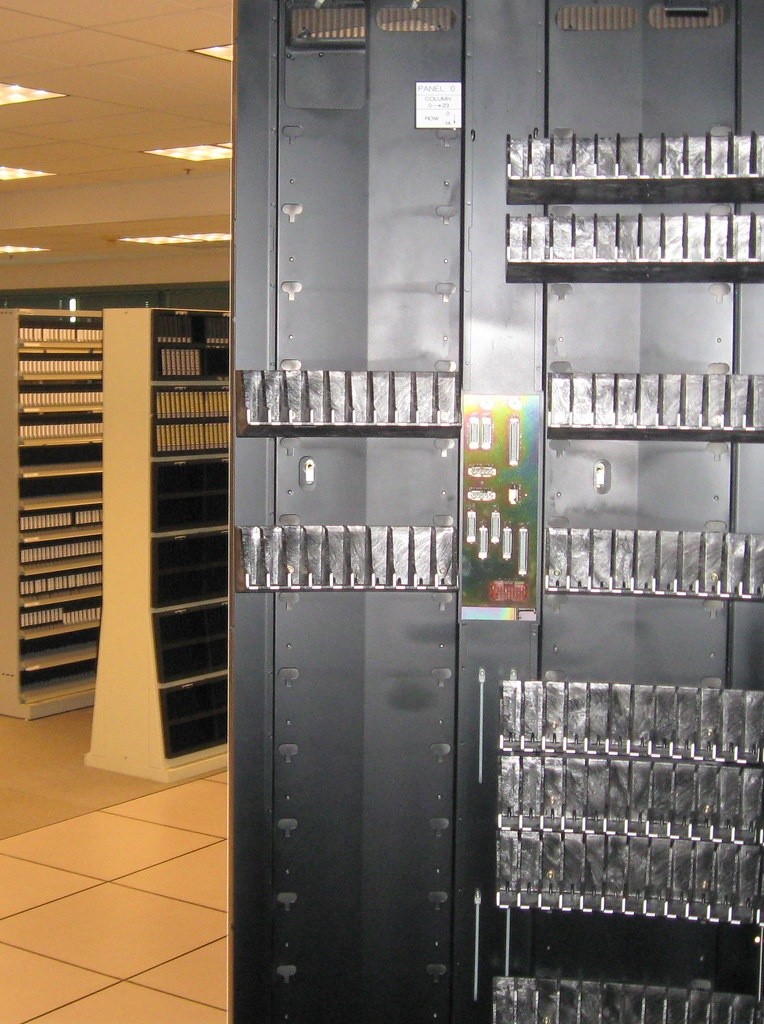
[156, 315, 229, 452]
[19, 328, 103, 626]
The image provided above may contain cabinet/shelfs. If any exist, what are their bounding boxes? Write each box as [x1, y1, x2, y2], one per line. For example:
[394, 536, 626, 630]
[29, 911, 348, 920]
[2, 302, 228, 784]
[240, 119, 764, 1024]
